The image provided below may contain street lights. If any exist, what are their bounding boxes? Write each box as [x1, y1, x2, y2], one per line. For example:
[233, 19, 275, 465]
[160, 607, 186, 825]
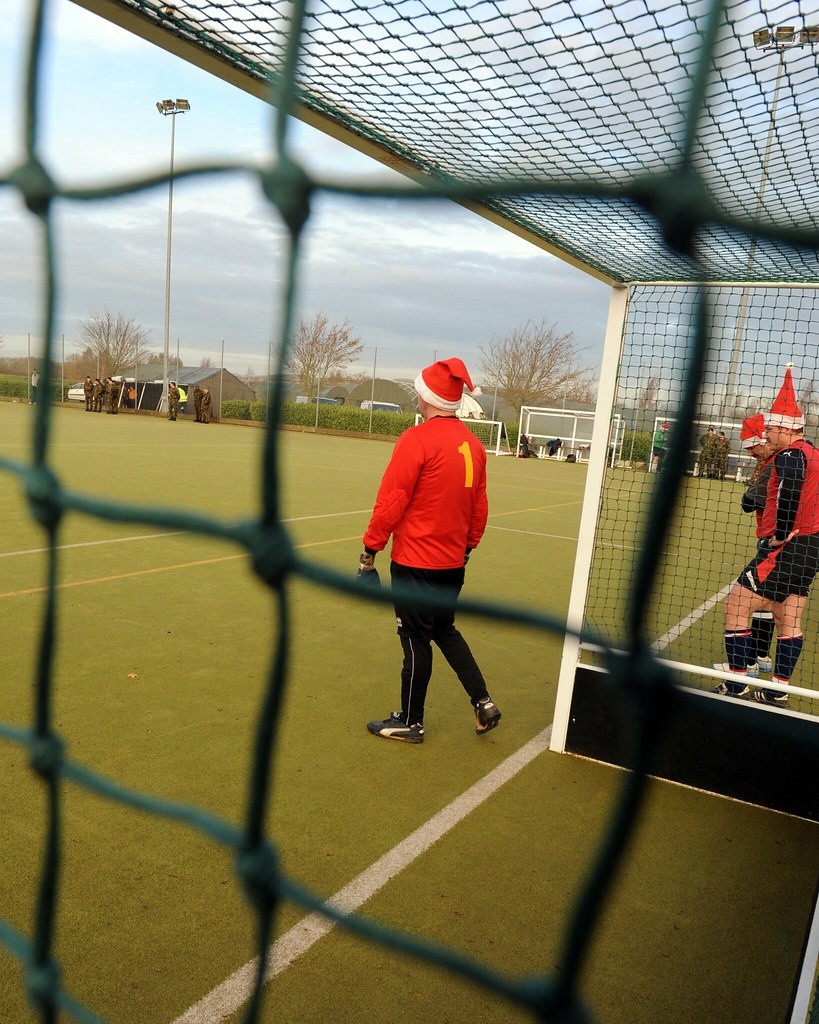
[718, 25, 819, 439]
[155, 99, 192, 415]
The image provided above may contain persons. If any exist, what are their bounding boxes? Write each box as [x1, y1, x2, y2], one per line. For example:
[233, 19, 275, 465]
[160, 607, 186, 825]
[653, 423, 671, 473]
[698, 428, 730, 481]
[30, 369, 41, 405]
[168, 382, 180, 421]
[84, 376, 135, 414]
[357, 357, 501, 744]
[711, 361, 819, 708]
[193, 386, 211, 423]
[467, 412, 487, 420]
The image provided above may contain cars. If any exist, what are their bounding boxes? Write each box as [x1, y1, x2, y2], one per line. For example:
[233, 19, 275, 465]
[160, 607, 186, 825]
[313, 397, 338, 404]
[67, 383, 96, 402]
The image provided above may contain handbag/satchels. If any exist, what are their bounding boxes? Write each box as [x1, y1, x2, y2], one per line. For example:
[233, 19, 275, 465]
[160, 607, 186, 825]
[565, 454, 576, 462]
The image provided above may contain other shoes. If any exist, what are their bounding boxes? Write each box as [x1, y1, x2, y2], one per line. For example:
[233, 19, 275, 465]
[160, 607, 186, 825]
[652, 469, 661, 473]
[168, 416, 176, 421]
[107, 408, 119, 414]
[85, 408, 92, 411]
[92, 409, 101, 412]
[199, 420, 209, 424]
[193, 418, 202, 422]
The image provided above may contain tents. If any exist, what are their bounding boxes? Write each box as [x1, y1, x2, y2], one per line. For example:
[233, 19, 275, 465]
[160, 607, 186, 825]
[250, 378, 519, 424]
[102, 363, 257, 415]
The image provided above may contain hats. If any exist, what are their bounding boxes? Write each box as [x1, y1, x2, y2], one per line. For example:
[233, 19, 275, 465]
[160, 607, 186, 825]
[661, 422, 669, 431]
[739, 414, 767, 448]
[764, 362, 804, 429]
[415, 358, 483, 411]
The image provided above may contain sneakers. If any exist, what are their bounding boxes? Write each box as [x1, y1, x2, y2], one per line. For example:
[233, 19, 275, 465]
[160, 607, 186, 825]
[474, 696, 501, 735]
[713, 662, 759, 678]
[756, 656, 773, 673]
[367, 711, 425, 744]
[710, 681, 789, 708]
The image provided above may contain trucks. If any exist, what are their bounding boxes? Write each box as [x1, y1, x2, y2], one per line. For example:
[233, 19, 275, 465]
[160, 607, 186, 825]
[361, 400, 401, 414]
